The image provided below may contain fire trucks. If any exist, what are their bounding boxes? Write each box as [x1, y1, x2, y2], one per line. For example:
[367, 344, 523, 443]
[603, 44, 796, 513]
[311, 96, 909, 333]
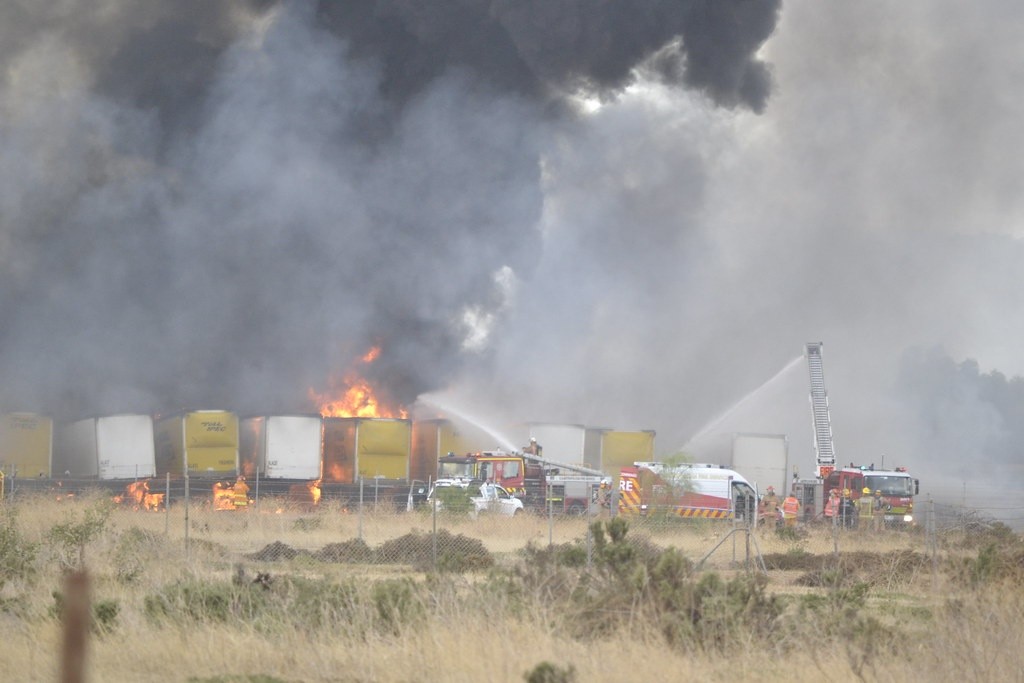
[438, 447, 612, 518]
[805, 341, 921, 530]
[600, 461, 786, 528]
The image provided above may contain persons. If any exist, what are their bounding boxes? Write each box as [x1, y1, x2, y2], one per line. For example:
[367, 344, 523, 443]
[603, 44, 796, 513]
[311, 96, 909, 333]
[529, 438, 538, 464]
[782, 492, 800, 527]
[761, 486, 779, 529]
[233, 475, 250, 514]
[824, 487, 891, 531]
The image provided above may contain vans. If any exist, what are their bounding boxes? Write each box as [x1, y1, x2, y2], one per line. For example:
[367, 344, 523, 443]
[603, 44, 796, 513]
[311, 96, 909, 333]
[407, 474, 525, 519]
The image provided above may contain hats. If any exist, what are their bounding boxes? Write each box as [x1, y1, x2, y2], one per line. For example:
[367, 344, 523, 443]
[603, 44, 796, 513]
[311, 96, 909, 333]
[875, 489, 882, 493]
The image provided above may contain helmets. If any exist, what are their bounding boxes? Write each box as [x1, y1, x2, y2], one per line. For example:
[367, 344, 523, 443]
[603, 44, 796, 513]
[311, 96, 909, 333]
[863, 487, 871, 494]
[833, 488, 840, 494]
[843, 489, 850, 495]
[766, 486, 774, 490]
